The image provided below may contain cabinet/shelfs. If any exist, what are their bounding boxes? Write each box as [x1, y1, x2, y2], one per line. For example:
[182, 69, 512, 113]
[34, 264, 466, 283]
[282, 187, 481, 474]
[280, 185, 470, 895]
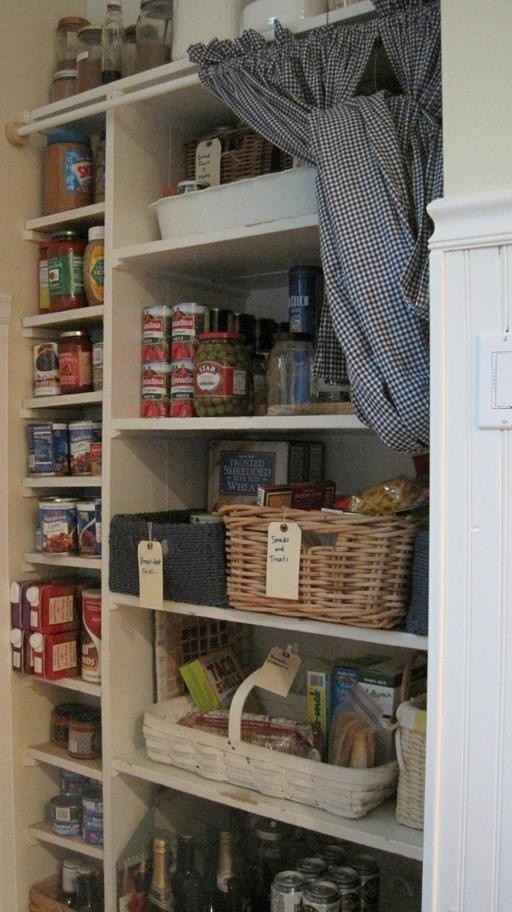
[0, 2, 448, 911]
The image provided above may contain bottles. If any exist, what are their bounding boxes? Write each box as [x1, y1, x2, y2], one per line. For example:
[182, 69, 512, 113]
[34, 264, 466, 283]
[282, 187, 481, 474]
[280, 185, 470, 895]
[85, 225, 108, 304]
[41, 130, 95, 217]
[49, 1, 170, 104]
[80, 588, 102, 686]
[38, 243, 51, 313]
[291, 267, 323, 333]
[143, 815, 290, 911]
[193, 332, 254, 415]
[267, 331, 316, 416]
[58, 856, 100, 911]
[94, 126, 108, 204]
[49, 231, 84, 311]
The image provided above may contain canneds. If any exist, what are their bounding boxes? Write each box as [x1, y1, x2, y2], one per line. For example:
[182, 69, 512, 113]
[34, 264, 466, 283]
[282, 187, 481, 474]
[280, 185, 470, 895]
[270, 844, 379, 911]
[24, 0, 289, 560]
[50, 702, 102, 848]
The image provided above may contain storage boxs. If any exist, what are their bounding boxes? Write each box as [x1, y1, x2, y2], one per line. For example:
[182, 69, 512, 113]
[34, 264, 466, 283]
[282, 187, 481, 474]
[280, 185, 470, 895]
[147, 162, 323, 242]
